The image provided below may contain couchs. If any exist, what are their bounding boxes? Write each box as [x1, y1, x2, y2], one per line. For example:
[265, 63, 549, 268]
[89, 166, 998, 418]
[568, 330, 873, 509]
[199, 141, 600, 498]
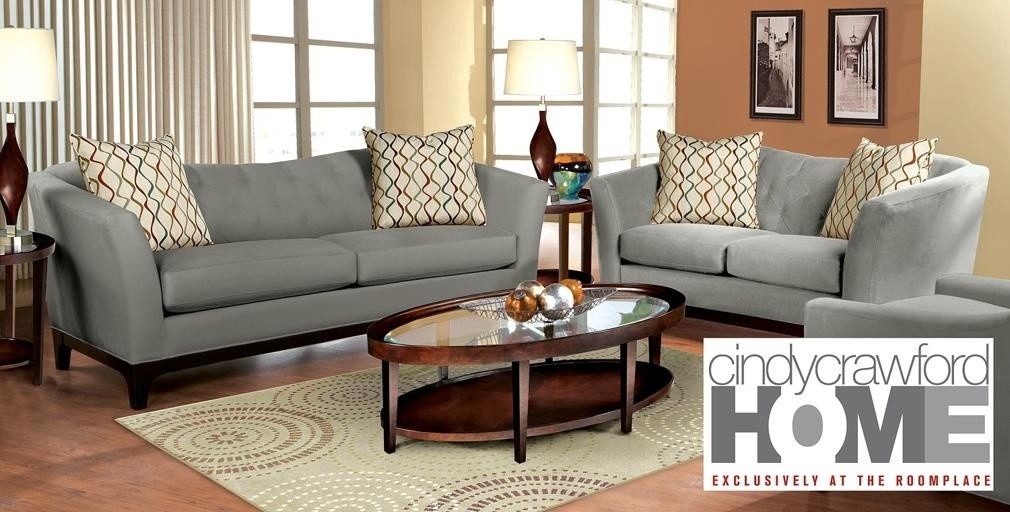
[18, 145, 549, 414]
[583, 136, 994, 334]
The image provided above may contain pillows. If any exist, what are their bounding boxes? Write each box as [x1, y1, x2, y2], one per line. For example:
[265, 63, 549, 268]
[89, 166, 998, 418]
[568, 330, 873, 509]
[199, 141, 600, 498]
[652, 127, 766, 231]
[357, 119, 494, 230]
[70, 132, 215, 252]
[821, 134, 940, 238]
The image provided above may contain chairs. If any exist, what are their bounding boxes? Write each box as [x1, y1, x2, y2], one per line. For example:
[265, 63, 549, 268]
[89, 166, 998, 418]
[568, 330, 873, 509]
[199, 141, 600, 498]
[795, 274, 1009, 504]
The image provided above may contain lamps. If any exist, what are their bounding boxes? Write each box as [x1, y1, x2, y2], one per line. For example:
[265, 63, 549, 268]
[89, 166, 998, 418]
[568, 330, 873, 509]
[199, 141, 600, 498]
[504, 37, 584, 182]
[0, 27, 59, 250]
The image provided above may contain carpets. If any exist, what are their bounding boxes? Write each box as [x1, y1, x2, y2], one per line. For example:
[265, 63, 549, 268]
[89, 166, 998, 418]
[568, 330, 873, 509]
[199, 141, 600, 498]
[106, 344, 706, 512]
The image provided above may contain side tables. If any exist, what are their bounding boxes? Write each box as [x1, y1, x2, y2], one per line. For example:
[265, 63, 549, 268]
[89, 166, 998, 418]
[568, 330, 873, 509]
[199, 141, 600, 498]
[540, 186, 595, 289]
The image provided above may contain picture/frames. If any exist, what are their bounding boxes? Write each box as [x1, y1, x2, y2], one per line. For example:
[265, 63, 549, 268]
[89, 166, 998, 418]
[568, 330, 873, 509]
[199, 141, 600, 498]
[749, 6, 890, 128]
